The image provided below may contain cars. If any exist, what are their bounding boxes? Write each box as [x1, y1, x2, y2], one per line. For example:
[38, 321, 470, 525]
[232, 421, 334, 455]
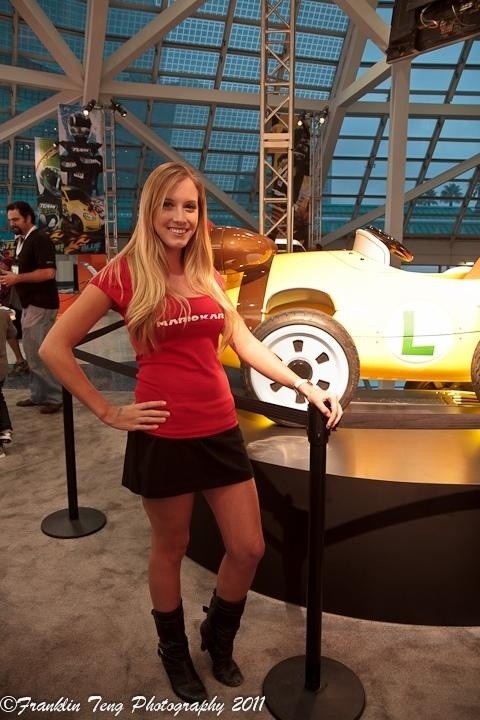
[38, 195, 59, 231]
[207, 218, 479, 429]
[61, 183, 105, 235]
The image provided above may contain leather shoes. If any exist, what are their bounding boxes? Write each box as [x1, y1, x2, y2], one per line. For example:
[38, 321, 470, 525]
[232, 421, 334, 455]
[39, 402, 63, 415]
[16, 399, 39, 407]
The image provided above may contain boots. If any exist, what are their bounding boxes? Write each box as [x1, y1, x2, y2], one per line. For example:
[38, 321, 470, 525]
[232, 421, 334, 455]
[149, 599, 209, 704]
[197, 586, 247, 687]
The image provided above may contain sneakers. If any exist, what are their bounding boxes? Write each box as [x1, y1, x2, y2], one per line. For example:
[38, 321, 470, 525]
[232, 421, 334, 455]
[6, 357, 32, 378]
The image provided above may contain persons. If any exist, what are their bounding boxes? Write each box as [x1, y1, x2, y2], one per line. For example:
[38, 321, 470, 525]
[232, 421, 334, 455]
[0, 201, 64, 414]
[38, 160, 346, 706]
[0, 302, 29, 460]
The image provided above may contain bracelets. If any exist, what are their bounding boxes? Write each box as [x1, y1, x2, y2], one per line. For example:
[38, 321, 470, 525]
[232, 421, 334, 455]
[294, 378, 314, 390]
